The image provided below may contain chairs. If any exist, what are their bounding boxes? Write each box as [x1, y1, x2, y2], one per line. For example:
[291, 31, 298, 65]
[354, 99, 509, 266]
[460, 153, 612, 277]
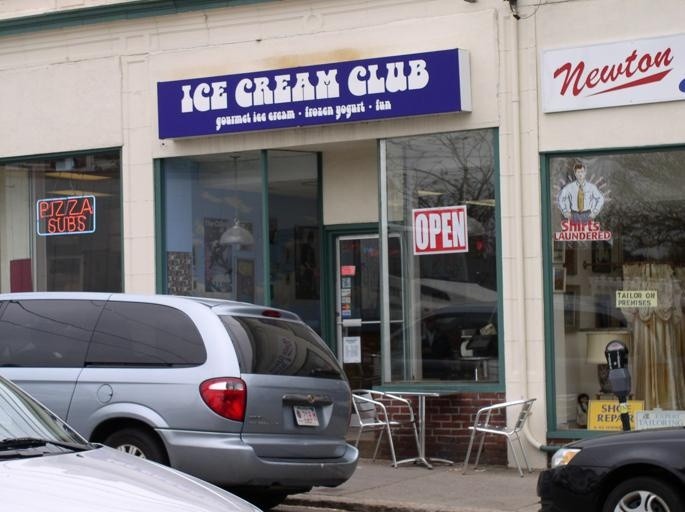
[461, 397, 537, 479]
[351, 389, 419, 468]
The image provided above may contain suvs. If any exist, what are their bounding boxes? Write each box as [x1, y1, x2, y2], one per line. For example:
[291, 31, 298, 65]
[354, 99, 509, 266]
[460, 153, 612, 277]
[0, 291, 359, 511]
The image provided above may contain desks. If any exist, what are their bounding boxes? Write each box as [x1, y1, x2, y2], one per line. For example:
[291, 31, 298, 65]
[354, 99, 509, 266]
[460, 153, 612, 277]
[373, 389, 456, 470]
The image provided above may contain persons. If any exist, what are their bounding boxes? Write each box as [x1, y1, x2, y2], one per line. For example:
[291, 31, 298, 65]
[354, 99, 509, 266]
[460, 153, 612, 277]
[558, 165, 605, 222]
[576, 393, 590, 428]
[422, 319, 450, 359]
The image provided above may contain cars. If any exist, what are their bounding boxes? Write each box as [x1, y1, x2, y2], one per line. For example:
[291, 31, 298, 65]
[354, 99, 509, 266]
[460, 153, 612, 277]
[377, 297, 665, 384]
[0, 374, 262, 512]
[536, 426, 685, 512]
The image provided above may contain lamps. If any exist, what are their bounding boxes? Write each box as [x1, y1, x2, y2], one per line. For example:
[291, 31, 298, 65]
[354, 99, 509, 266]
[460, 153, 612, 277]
[219, 154, 254, 246]
